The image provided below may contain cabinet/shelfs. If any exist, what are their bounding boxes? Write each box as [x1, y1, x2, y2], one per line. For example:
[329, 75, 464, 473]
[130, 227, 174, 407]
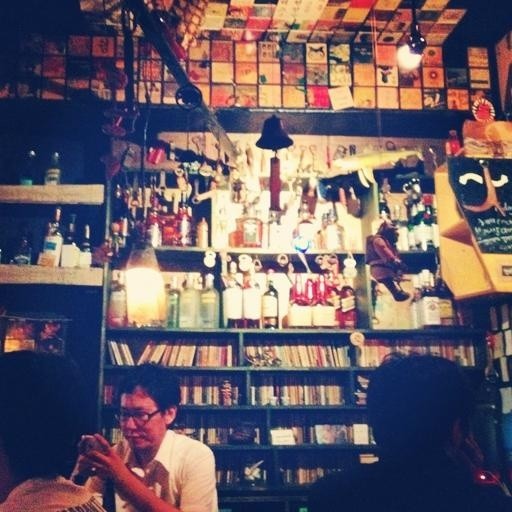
[1, 95, 512, 512]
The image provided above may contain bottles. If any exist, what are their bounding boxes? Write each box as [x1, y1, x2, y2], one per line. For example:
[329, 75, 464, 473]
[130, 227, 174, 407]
[236, 181, 344, 252]
[377, 178, 437, 251]
[410, 265, 477, 328]
[17, 143, 62, 186]
[107, 269, 129, 328]
[8, 201, 92, 269]
[166, 269, 357, 330]
[113, 183, 209, 247]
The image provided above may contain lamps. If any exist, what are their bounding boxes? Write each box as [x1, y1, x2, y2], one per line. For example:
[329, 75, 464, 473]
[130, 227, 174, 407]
[406, 0, 427, 55]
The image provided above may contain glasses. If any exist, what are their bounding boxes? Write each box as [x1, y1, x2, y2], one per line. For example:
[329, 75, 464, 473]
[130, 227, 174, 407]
[114, 409, 162, 424]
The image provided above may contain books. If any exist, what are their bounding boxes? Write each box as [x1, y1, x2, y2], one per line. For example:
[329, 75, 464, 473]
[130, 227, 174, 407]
[108, 336, 476, 485]
[488, 303, 510, 415]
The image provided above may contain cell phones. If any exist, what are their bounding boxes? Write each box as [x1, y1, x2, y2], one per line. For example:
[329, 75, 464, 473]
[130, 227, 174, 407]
[82, 436, 103, 456]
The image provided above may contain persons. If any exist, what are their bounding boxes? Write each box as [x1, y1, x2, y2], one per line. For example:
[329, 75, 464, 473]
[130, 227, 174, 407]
[69, 364, 219, 512]
[306, 352, 511, 512]
[366, 221, 411, 302]
[107, 222, 123, 258]
